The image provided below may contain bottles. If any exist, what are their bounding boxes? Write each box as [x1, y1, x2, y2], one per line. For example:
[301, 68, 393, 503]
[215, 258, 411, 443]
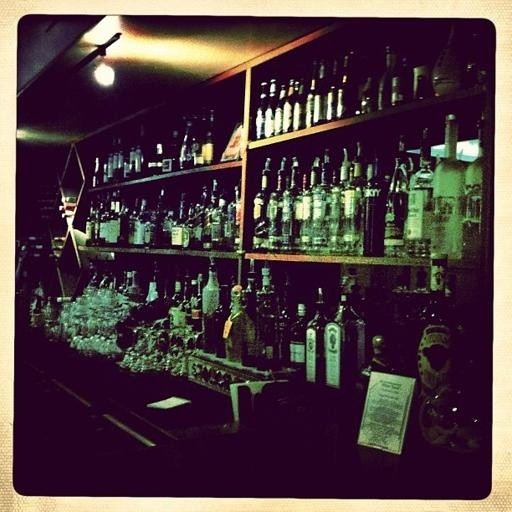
[85, 19, 495, 258]
[23, 194, 78, 259]
[115, 258, 485, 456]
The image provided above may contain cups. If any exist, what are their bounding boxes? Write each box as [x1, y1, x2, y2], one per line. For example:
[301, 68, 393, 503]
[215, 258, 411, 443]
[26, 273, 136, 361]
